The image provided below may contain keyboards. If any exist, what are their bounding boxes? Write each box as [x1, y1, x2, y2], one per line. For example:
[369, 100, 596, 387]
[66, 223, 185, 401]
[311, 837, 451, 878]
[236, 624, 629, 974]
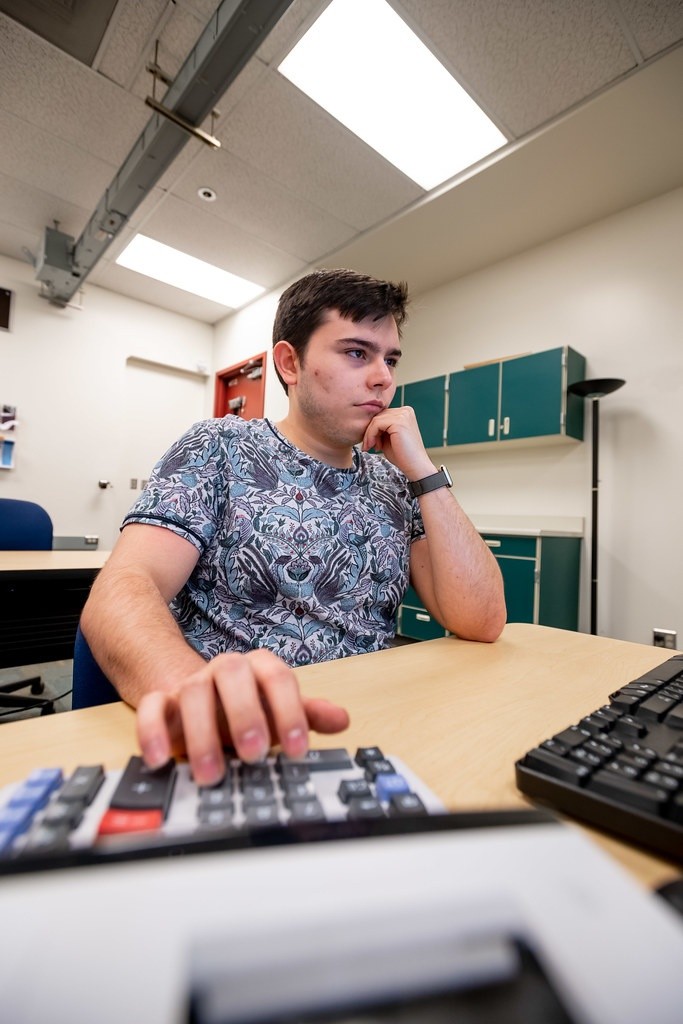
[515, 654, 683, 865]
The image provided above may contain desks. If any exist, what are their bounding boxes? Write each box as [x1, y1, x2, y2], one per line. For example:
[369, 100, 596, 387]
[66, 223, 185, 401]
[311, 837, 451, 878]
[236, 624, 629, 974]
[0, 550, 113, 581]
[0, 622, 683, 890]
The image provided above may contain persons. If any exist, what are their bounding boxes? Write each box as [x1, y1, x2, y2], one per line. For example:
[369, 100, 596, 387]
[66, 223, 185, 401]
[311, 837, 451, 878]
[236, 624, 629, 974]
[80, 266, 506, 788]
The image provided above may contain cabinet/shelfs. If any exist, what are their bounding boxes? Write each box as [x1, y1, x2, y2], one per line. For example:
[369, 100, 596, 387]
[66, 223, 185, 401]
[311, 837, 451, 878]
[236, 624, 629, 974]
[395, 529, 581, 642]
[362, 346, 586, 460]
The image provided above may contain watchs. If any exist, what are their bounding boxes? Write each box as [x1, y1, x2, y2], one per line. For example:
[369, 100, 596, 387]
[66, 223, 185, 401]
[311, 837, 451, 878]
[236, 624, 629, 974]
[407, 465, 452, 500]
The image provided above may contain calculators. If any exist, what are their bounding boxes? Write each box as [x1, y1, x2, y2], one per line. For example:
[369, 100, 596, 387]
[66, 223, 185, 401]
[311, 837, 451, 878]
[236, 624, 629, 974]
[0, 738, 437, 874]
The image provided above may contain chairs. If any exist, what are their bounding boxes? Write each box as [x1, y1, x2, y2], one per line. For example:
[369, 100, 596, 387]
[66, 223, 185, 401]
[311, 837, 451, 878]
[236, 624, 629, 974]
[0, 498, 58, 716]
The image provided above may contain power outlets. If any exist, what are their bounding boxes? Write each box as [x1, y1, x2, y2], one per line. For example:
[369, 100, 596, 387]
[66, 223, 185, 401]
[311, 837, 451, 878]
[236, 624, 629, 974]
[652, 628, 677, 650]
